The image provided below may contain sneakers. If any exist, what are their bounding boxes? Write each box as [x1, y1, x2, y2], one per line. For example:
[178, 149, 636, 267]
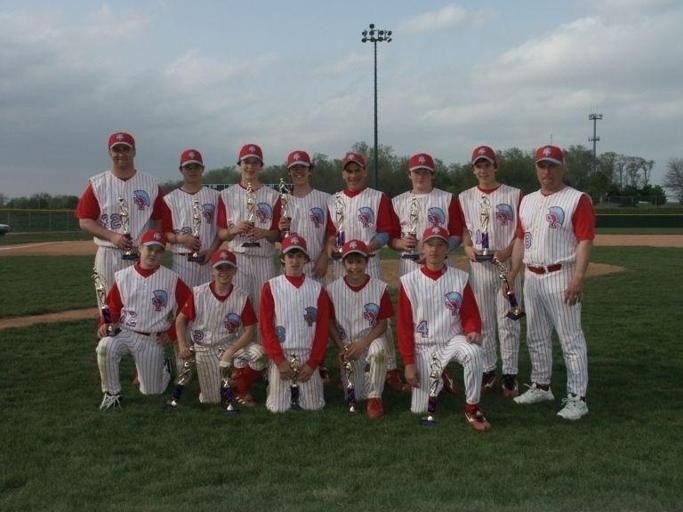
[480, 377, 498, 394]
[164, 357, 174, 381]
[235, 392, 256, 409]
[502, 379, 519, 398]
[513, 382, 555, 405]
[386, 369, 410, 392]
[366, 398, 383, 420]
[321, 365, 331, 385]
[556, 392, 589, 421]
[131, 372, 140, 387]
[99, 392, 122, 413]
[442, 369, 459, 395]
[463, 408, 491, 432]
[339, 377, 345, 390]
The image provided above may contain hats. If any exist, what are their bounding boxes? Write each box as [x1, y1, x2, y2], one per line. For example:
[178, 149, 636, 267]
[340, 151, 366, 172]
[287, 150, 312, 170]
[180, 149, 204, 168]
[535, 145, 563, 166]
[238, 143, 264, 163]
[210, 249, 238, 269]
[422, 225, 450, 245]
[471, 146, 496, 165]
[280, 234, 307, 254]
[340, 239, 369, 258]
[108, 132, 135, 151]
[407, 153, 435, 172]
[138, 227, 167, 250]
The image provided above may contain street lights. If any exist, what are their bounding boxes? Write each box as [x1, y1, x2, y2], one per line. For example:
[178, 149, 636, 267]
[361, 23, 392, 189]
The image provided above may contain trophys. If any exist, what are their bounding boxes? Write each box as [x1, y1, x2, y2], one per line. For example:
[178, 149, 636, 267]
[420, 354, 441, 425]
[289, 353, 301, 410]
[492, 257, 524, 320]
[90, 267, 114, 337]
[402, 194, 420, 258]
[188, 202, 205, 262]
[117, 196, 137, 259]
[241, 182, 262, 247]
[342, 343, 361, 417]
[165, 343, 195, 408]
[277, 177, 290, 242]
[330, 193, 346, 257]
[216, 347, 239, 414]
[474, 192, 493, 260]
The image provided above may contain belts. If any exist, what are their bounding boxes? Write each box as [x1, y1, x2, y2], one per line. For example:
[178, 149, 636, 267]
[241, 242, 261, 247]
[174, 252, 194, 257]
[133, 329, 164, 339]
[525, 262, 563, 275]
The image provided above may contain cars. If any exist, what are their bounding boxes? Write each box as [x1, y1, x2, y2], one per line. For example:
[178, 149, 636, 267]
[0, 224, 10, 236]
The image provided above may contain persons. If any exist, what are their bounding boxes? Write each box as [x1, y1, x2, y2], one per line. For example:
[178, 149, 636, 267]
[94, 228, 191, 410]
[174, 250, 268, 408]
[325, 154, 411, 392]
[279, 151, 338, 283]
[161, 150, 229, 291]
[501, 145, 597, 421]
[397, 229, 491, 431]
[387, 155, 466, 277]
[77, 132, 172, 327]
[324, 240, 395, 420]
[261, 236, 332, 412]
[219, 145, 283, 379]
[458, 146, 525, 397]
